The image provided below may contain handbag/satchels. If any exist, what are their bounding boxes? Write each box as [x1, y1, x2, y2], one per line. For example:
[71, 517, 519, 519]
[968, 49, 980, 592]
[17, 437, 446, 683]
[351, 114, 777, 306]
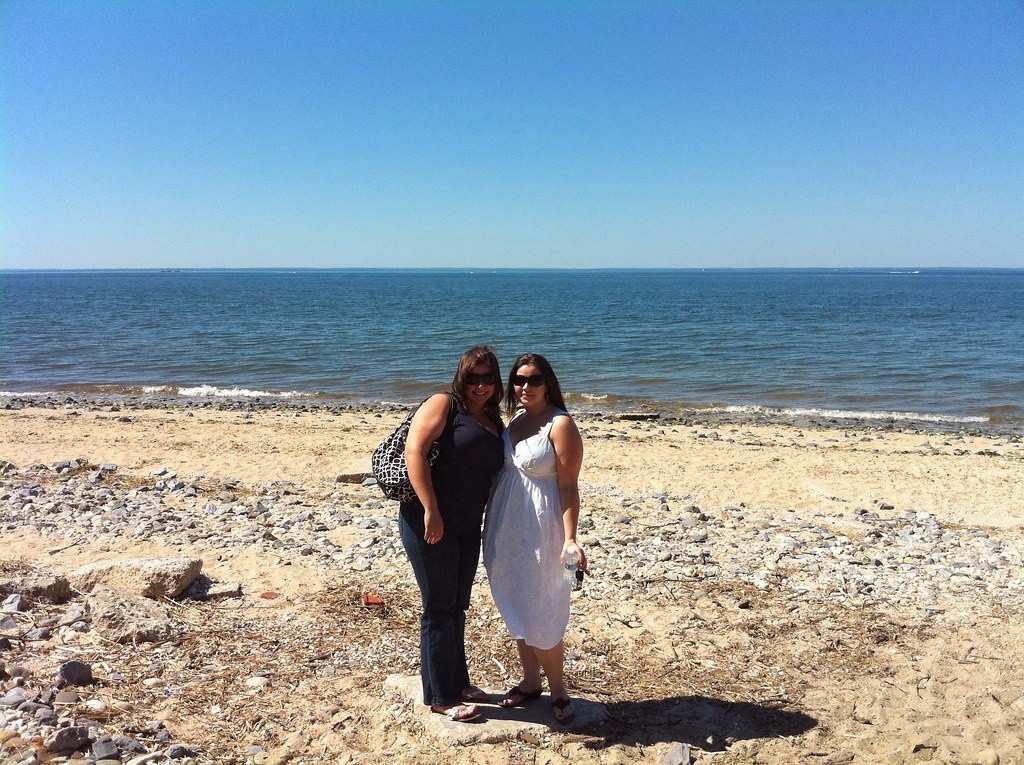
[372, 393, 454, 502]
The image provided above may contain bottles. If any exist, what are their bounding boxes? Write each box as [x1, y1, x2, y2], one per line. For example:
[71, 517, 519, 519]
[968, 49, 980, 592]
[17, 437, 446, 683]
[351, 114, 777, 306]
[564, 546, 580, 585]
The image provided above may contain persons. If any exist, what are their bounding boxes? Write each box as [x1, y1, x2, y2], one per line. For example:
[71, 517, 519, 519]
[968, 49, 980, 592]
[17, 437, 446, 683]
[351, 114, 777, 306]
[482, 354, 587, 724]
[398, 346, 506, 722]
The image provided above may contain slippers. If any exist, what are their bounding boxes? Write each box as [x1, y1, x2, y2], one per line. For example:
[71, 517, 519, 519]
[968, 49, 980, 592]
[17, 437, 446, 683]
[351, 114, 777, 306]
[552, 698, 577, 722]
[460, 686, 490, 702]
[431, 702, 482, 721]
[496, 686, 543, 707]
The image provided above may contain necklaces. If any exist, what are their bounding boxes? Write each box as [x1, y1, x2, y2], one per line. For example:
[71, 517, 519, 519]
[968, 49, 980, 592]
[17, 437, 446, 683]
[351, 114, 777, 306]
[520, 405, 552, 439]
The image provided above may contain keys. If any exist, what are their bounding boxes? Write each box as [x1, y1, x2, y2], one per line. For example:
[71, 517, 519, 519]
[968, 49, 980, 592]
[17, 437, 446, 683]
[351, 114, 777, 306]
[576, 566, 584, 590]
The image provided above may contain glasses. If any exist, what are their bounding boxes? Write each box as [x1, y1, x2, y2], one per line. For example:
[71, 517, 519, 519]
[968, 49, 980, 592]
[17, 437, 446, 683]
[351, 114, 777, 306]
[514, 375, 545, 386]
[466, 372, 496, 385]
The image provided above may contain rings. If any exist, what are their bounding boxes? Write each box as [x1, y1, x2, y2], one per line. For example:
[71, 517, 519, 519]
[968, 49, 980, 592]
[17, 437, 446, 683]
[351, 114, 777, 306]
[431, 531, 435, 535]
